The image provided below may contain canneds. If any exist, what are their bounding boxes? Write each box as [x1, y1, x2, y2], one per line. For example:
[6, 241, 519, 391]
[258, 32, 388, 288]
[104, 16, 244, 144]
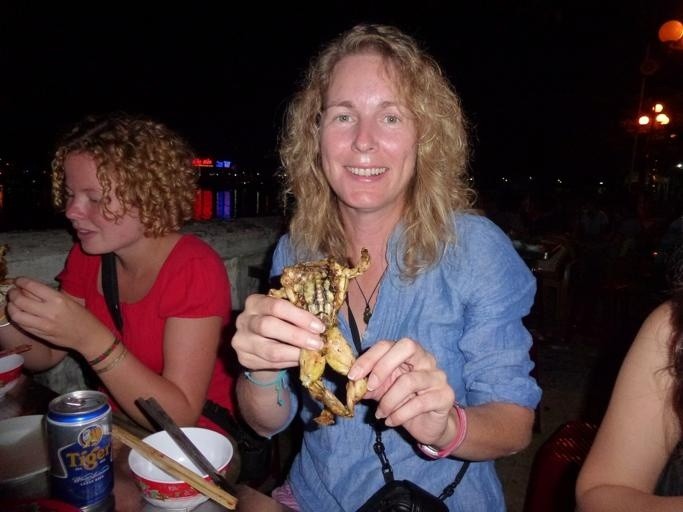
[43, 389, 116, 509]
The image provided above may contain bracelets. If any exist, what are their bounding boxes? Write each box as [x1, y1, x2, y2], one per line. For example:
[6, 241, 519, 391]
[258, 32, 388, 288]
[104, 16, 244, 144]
[0, 314, 11, 328]
[86, 335, 119, 364]
[242, 370, 291, 404]
[416, 400, 468, 459]
[93, 347, 127, 375]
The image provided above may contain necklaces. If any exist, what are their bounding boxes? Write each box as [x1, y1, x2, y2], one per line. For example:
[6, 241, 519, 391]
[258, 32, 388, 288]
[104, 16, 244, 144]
[346, 257, 387, 326]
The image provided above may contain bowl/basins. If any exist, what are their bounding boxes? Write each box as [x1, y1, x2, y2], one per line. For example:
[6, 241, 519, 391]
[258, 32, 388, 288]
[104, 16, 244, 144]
[0, 354, 25, 399]
[127, 426, 235, 512]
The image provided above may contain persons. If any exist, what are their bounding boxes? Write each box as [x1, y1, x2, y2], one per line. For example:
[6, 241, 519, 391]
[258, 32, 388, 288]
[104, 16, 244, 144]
[573, 267, 681, 511]
[0, 113, 245, 485]
[229, 21, 543, 511]
[267, 194, 329, 292]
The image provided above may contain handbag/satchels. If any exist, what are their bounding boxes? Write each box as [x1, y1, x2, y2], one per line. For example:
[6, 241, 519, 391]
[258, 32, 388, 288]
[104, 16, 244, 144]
[355, 479, 449, 512]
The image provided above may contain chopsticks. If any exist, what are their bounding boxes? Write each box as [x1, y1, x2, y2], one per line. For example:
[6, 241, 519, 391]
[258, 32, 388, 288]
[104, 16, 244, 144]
[111, 424, 238, 511]
[0, 344, 31, 358]
[139, 394, 238, 497]
